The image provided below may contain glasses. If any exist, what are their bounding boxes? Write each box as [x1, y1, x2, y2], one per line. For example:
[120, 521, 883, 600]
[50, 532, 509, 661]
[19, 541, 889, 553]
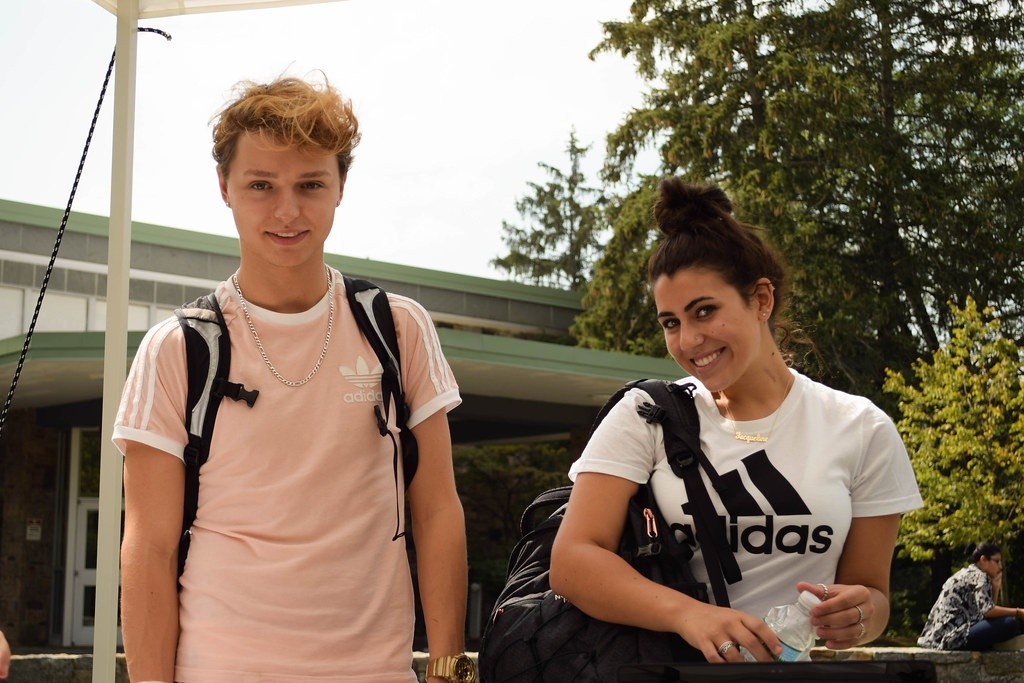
[986, 556, 1003, 564]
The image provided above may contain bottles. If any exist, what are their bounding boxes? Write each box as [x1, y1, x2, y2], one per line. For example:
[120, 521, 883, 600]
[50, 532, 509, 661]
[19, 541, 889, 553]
[741, 589, 821, 662]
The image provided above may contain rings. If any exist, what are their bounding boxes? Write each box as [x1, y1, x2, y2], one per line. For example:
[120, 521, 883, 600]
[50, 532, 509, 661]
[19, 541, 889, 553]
[716, 639, 735, 656]
[815, 583, 829, 602]
[855, 622, 866, 640]
[853, 604, 863, 625]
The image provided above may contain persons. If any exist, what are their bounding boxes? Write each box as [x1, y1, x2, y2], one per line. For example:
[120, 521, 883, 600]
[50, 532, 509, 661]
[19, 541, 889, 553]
[916, 542, 1024, 652]
[546, 176, 930, 663]
[109, 77, 484, 683]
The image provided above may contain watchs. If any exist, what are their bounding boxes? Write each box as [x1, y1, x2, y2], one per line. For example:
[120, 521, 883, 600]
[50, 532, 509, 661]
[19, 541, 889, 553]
[1016, 607, 1020, 617]
[423, 651, 479, 683]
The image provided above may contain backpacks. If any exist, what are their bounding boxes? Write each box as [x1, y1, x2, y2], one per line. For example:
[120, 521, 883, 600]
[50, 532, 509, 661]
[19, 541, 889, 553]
[472, 379, 746, 683]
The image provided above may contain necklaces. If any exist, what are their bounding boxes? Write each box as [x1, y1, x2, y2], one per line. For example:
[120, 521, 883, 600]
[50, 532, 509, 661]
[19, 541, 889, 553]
[717, 371, 792, 444]
[232, 256, 335, 388]
